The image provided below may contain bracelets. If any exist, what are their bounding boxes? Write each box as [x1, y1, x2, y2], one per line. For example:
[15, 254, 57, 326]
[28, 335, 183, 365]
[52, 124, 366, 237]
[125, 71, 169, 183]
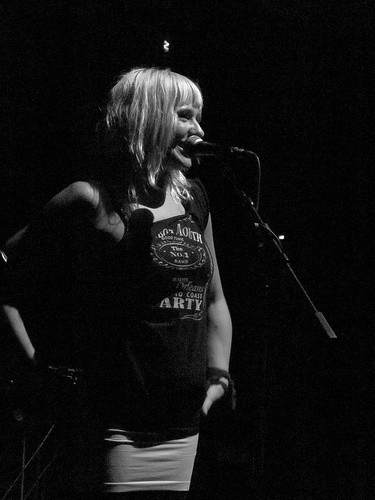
[207, 365, 231, 395]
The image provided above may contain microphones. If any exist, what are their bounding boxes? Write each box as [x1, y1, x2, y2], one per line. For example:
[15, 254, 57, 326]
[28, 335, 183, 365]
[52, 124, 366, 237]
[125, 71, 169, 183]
[182, 135, 257, 158]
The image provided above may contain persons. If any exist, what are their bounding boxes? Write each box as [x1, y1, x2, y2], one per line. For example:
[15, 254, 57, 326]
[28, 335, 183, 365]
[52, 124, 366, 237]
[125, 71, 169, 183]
[0, 67, 234, 493]
